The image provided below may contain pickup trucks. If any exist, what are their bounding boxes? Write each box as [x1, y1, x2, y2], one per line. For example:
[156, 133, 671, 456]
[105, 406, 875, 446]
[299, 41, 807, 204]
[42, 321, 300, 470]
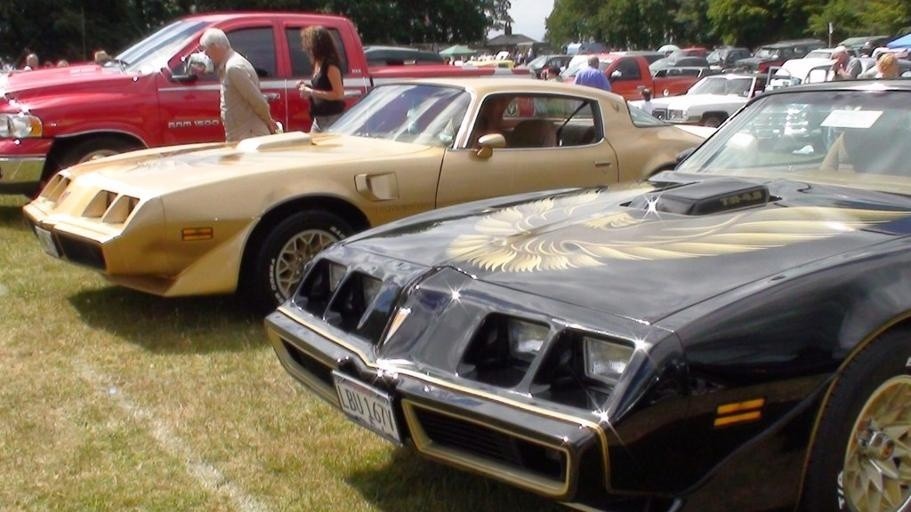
[0, 12, 537, 201]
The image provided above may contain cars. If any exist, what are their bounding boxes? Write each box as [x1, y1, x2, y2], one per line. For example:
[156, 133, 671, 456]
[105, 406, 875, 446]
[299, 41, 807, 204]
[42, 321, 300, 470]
[22, 76, 718, 315]
[263, 78, 911, 512]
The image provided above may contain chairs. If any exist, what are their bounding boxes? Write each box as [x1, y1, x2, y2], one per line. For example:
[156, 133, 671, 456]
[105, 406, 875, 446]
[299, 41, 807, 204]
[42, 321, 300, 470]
[819, 119, 911, 177]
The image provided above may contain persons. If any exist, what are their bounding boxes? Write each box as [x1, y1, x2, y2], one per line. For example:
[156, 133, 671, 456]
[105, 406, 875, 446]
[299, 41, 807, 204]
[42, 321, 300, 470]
[877, 53, 902, 79]
[572, 57, 612, 94]
[20, 48, 114, 70]
[830, 45, 860, 82]
[296, 25, 345, 135]
[441, 53, 476, 69]
[545, 65, 563, 84]
[203, 28, 278, 144]
